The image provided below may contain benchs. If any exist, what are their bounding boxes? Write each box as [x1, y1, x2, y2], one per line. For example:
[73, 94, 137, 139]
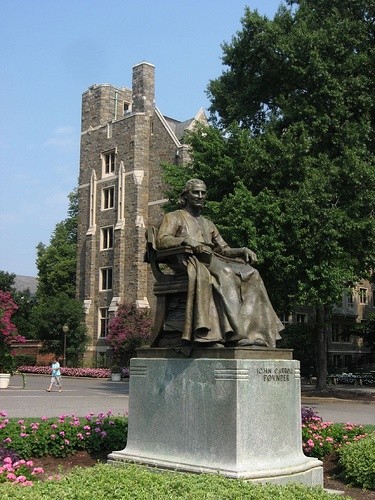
[352, 377, 366, 386]
[308, 375, 338, 386]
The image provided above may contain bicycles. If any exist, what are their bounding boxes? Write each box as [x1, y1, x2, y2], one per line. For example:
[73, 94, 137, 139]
[320, 362, 375, 385]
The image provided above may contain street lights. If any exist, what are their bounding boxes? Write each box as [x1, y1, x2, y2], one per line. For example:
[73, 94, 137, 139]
[62, 323, 69, 366]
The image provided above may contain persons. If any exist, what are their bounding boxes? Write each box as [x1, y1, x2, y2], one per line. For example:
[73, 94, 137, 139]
[155, 178, 286, 350]
[46, 357, 63, 392]
